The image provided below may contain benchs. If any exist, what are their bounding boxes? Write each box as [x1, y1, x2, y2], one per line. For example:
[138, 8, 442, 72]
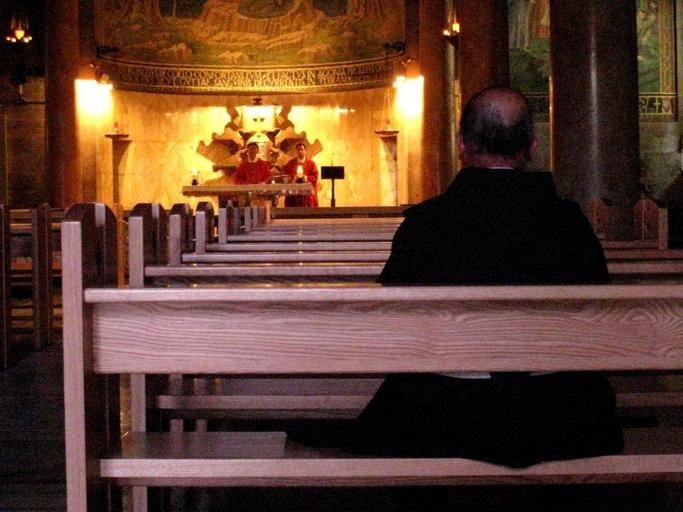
[3, 200, 683, 512]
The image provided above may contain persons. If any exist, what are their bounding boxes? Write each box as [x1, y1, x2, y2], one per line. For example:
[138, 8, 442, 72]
[356, 85, 625, 469]
[234, 142, 319, 208]
